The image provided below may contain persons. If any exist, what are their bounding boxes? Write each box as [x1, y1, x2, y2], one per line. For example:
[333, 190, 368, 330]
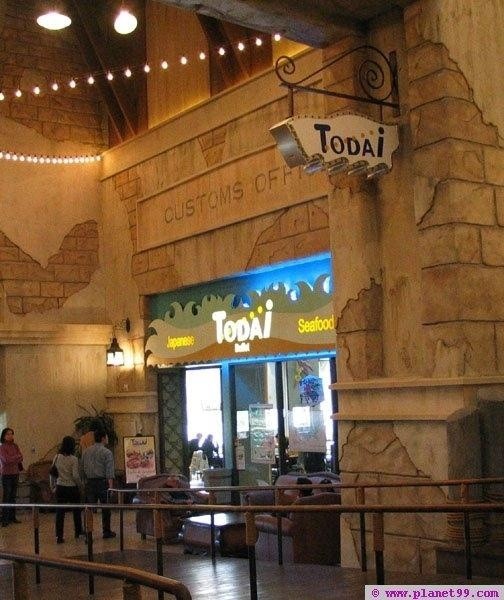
[318, 478, 335, 493]
[49, 436, 87, 544]
[199, 434, 219, 479]
[80, 430, 117, 544]
[0, 428, 23, 528]
[271, 477, 315, 517]
[188, 433, 203, 467]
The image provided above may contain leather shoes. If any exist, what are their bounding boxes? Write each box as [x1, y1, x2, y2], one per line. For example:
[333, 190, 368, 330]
[102, 531, 116, 539]
[55, 536, 65, 544]
[74, 531, 97, 546]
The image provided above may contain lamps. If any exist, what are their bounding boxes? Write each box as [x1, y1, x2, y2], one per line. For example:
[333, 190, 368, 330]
[106, 338, 124, 367]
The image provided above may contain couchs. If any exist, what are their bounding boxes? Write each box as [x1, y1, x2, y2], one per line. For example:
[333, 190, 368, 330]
[244, 471, 339, 566]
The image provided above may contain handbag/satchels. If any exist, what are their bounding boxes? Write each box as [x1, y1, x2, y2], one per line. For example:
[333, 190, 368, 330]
[48, 462, 59, 480]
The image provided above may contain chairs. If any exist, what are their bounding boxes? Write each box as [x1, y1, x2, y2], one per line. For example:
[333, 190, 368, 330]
[136, 473, 216, 544]
